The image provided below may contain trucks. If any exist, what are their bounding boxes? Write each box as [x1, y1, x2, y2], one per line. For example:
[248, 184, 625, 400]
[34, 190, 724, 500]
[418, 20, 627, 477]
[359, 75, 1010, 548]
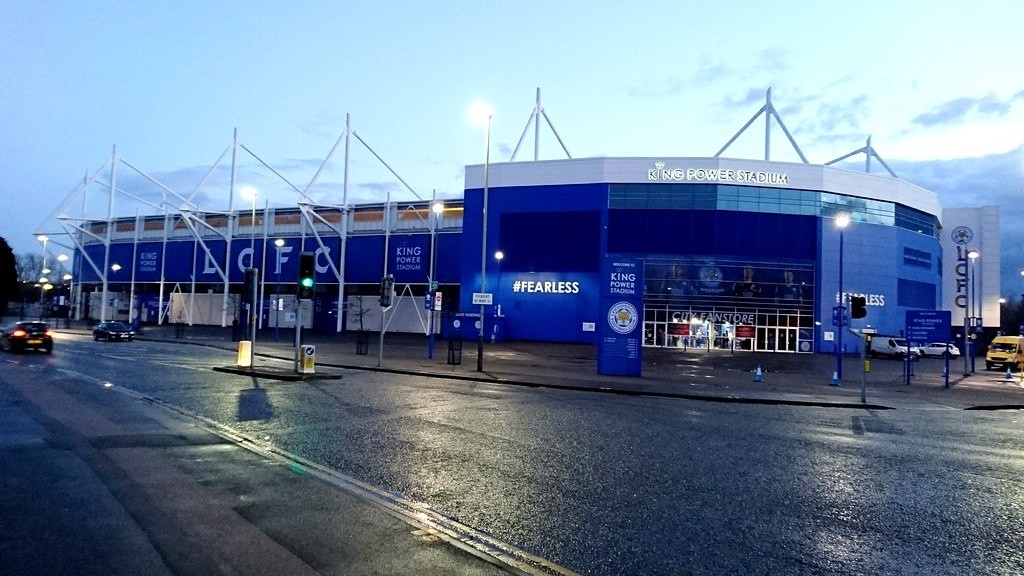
[871, 336, 920, 362]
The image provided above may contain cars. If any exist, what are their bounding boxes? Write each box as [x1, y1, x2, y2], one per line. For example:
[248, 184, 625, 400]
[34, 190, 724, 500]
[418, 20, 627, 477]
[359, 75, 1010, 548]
[92, 320, 136, 342]
[0, 321, 54, 354]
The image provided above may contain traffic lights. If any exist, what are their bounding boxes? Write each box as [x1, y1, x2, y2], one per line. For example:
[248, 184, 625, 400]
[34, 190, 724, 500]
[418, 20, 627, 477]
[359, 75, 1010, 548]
[378, 276, 394, 307]
[242, 267, 261, 299]
[297, 252, 316, 302]
[849, 295, 868, 319]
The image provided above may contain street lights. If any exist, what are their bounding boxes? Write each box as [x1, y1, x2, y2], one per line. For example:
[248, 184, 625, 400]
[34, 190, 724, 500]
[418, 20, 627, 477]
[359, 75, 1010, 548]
[967, 249, 980, 373]
[833, 214, 850, 380]
[428, 202, 445, 357]
[111, 263, 121, 321]
[474, 104, 499, 373]
[36, 233, 49, 320]
[242, 189, 259, 270]
[998, 298, 1006, 335]
[274, 239, 285, 343]
[54, 255, 69, 328]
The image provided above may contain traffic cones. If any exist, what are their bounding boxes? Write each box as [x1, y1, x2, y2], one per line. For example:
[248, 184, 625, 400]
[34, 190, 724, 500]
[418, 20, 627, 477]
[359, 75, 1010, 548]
[753, 362, 766, 382]
[942, 360, 950, 377]
[1004, 366, 1013, 379]
[827, 369, 841, 387]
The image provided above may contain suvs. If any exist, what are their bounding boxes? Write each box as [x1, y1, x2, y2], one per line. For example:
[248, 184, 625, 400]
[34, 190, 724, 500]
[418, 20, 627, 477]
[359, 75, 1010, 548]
[918, 342, 961, 360]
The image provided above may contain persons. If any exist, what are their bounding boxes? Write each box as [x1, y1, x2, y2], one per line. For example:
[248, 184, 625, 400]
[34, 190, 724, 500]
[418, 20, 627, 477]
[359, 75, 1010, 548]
[670, 263, 697, 295]
[733, 265, 761, 297]
[707, 269, 717, 281]
[775, 269, 804, 304]
[658, 322, 732, 348]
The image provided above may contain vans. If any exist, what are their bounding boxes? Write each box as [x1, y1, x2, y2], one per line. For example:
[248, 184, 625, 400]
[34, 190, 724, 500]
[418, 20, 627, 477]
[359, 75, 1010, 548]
[985, 334, 1024, 372]
